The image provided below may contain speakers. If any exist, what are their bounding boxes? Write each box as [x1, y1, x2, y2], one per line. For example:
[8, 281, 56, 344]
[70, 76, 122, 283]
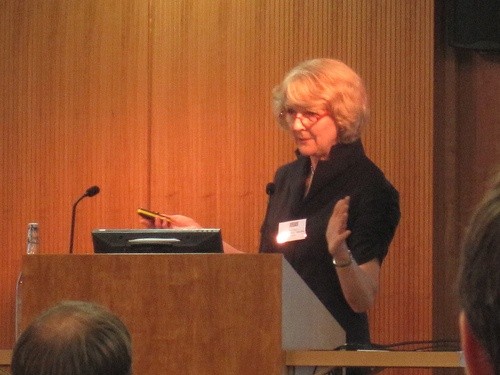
[447, 0, 500, 50]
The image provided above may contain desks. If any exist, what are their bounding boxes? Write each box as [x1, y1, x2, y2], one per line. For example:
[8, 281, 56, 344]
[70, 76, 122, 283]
[284, 349, 466, 375]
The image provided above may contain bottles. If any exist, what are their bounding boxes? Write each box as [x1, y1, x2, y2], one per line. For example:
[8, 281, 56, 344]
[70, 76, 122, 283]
[15, 223, 40, 343]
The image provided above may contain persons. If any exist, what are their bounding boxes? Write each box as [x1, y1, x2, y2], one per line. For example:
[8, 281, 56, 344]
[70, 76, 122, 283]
[10, 300, 134, 375]
[457, 177, 500, 375]
[140, 59, 400, 375]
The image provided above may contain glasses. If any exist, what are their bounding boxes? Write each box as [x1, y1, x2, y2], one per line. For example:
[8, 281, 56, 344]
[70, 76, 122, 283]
[278, 109, 331, 129]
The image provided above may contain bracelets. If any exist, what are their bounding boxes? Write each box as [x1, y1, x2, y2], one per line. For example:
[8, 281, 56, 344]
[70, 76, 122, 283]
[331, 250, 353, 269]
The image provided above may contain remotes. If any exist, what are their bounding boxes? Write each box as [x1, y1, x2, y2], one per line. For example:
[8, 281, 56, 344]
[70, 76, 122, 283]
[137, 206, 172, 223]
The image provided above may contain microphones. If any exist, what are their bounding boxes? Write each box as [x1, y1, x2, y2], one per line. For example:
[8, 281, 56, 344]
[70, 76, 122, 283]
[261, 182, 275, 253]
[70, 185, 99, 253]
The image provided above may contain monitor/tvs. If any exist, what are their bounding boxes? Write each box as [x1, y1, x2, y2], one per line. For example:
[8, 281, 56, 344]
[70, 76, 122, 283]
[92, 227, 224, 254]
